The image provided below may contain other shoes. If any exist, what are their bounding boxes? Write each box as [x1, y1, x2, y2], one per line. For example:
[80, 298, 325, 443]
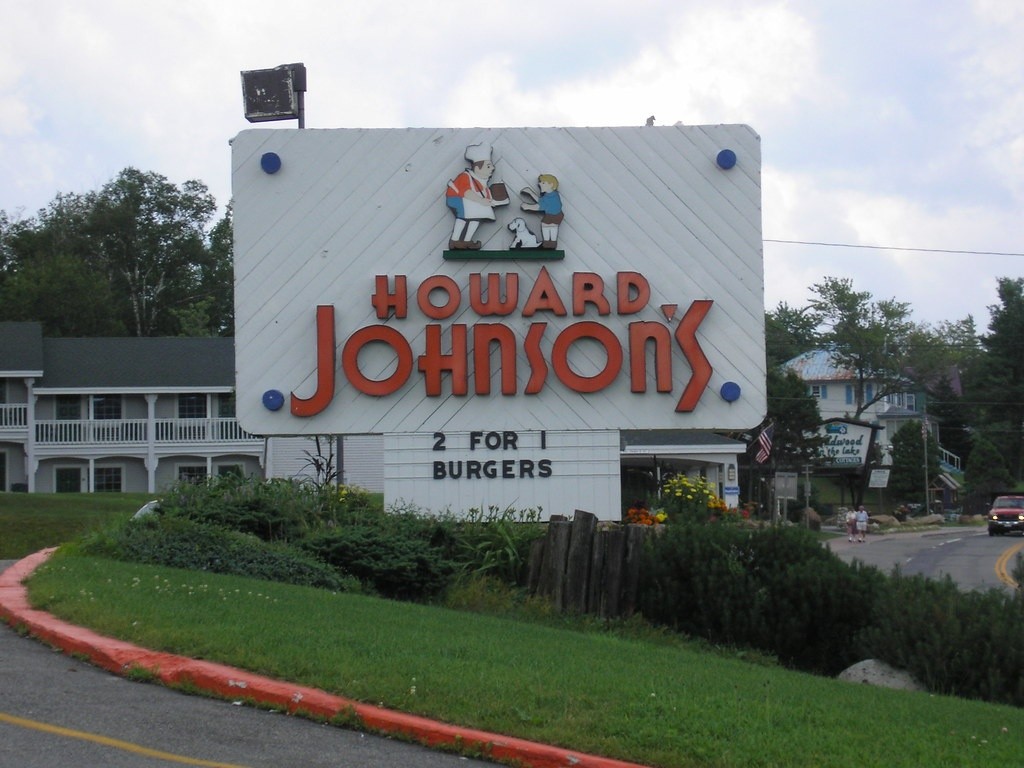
[858, 539, 861, 542]
[863, 540, 865, 542]
[849, 540, 852, 542]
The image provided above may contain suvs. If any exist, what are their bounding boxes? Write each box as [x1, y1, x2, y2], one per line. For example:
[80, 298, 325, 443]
[986, 495, 1024, 537]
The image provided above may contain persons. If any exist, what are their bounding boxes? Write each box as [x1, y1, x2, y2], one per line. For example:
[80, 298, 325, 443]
[846, 506, 857, 542]
[856, 506, 869, 543]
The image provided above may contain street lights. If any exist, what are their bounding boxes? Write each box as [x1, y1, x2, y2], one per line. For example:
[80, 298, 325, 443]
[922, 408, 930, 515]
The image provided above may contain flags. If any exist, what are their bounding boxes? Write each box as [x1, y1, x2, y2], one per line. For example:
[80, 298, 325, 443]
[756, 422, 775, 464]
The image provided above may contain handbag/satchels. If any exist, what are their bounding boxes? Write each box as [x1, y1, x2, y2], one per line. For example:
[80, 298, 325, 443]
[847, 513, 852, 525]
